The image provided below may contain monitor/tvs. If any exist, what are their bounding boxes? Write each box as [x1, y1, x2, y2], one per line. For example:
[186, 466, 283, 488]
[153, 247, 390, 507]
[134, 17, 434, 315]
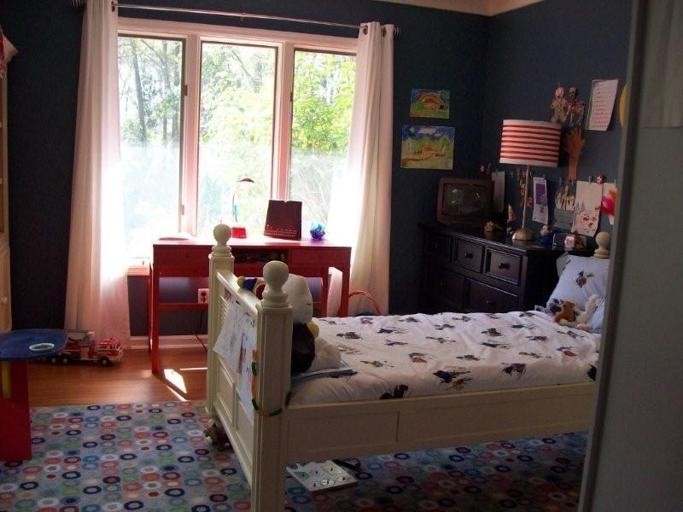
[437, 177, 496, 227]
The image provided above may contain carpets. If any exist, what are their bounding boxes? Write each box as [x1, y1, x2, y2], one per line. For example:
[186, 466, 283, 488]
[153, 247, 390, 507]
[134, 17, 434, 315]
[0, 399, 587, 511]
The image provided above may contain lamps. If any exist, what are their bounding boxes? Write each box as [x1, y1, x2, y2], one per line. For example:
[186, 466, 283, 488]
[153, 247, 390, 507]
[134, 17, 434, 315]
[498, 119, 561, 242]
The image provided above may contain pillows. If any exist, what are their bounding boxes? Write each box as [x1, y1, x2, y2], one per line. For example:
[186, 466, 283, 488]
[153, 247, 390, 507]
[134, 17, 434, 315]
[535, 252, 609, 333]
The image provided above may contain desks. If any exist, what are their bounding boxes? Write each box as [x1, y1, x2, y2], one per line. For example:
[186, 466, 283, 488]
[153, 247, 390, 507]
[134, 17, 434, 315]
[146, 235, 351, 374]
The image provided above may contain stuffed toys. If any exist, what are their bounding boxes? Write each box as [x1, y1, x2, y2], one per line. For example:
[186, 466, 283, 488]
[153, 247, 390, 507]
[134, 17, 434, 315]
[554, 294, 600, 331]
[282, 275, 341, 374]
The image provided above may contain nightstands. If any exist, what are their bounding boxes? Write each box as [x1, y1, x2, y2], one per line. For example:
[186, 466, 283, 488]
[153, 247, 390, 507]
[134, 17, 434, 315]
[421, 224, 594, 315]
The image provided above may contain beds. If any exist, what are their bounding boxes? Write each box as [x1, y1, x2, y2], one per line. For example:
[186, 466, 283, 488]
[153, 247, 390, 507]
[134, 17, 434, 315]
[205, 223, 612, 510]
